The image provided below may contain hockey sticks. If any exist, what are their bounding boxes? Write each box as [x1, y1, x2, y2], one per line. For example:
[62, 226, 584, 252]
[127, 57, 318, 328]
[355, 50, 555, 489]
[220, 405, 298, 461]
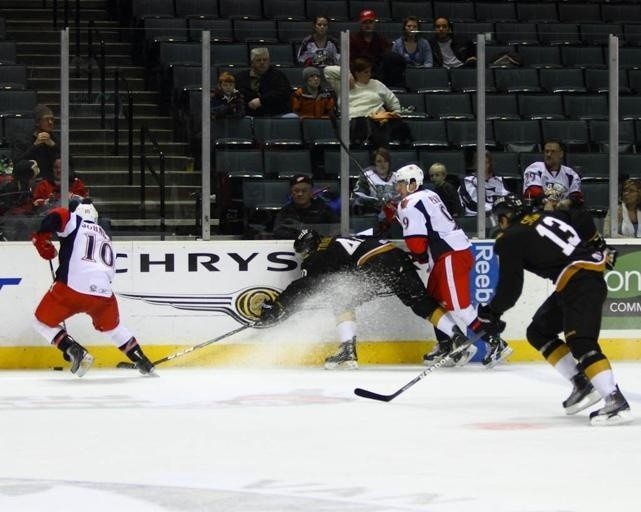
[117, 315, 264, 370]
[355, 329, 485, 400]
[328, 109, 398, 214]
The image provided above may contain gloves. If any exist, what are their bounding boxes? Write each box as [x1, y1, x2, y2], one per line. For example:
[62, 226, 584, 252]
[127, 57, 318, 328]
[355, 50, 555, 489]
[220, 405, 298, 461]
[31, 232, 57, 260]
[261, 299, 280, 322]
[594, 234, 617, 270]
[478, 302, 505, 335]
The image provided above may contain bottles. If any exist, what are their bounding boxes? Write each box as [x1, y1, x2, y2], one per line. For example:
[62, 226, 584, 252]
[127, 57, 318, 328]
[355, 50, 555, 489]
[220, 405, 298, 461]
[544, 183, 558, 207]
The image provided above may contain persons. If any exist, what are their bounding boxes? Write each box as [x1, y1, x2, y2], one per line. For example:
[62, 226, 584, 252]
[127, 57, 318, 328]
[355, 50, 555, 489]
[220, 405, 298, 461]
[324, 57, 403, 121]
[0, 153, 89, 216]
[211, 71, 248, 118]
[390, 16, 435, 69]
[291, 67, 336, 117]
[428, 162, 465, 217]
[31, 201, 155, 376]
[429, 15, 479, 69]
[11, 104, 77, 180]
[258, 226, 473, 367]
[457, 150, 516, 218]
[275, 173, 341, 225]
[349, 10, 391, 68]
[523, 138, 585, 212]
[298, 14, 341, 68]
[233, 47, 293, 117]
[381, 164, 483, 363]
[350, 146, 401, 215]
[603, 175, 641, 238]
[478, 194, 631, 420]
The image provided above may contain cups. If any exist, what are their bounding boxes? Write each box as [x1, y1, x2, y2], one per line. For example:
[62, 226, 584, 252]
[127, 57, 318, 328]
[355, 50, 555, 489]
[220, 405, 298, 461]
[525, 199, 536, 213]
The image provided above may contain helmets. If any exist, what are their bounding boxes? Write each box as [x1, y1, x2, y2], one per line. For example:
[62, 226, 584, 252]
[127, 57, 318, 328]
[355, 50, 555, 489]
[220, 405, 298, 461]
[294, 229, 319, 257]
[492, 196, 524, 223]
[73, 200, 100, 223]
[393, 163, 423, 192]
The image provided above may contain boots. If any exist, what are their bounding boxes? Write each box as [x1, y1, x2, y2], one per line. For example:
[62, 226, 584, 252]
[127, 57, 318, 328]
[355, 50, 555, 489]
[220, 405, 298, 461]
[423, 324, 507, 366]
[66, 334, 86, 373]
[562, 364, 592, 409]
[589, 384, 630, 419]
[131, 348, 154, 373]
[325, 337, 356, 363]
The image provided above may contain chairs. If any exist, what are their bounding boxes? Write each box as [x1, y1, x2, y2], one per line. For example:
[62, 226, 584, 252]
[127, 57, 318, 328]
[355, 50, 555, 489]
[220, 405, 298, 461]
[1, 2, 114, 238]
[126, 1, 641, 238]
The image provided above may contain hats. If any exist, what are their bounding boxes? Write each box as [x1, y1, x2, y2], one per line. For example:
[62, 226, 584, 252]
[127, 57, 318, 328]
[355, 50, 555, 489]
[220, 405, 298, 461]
[32, 103, 51, 120]
[360, 10, 375, 25]
[301, 66, 320, 82]
[291, 174, 314, 189]
